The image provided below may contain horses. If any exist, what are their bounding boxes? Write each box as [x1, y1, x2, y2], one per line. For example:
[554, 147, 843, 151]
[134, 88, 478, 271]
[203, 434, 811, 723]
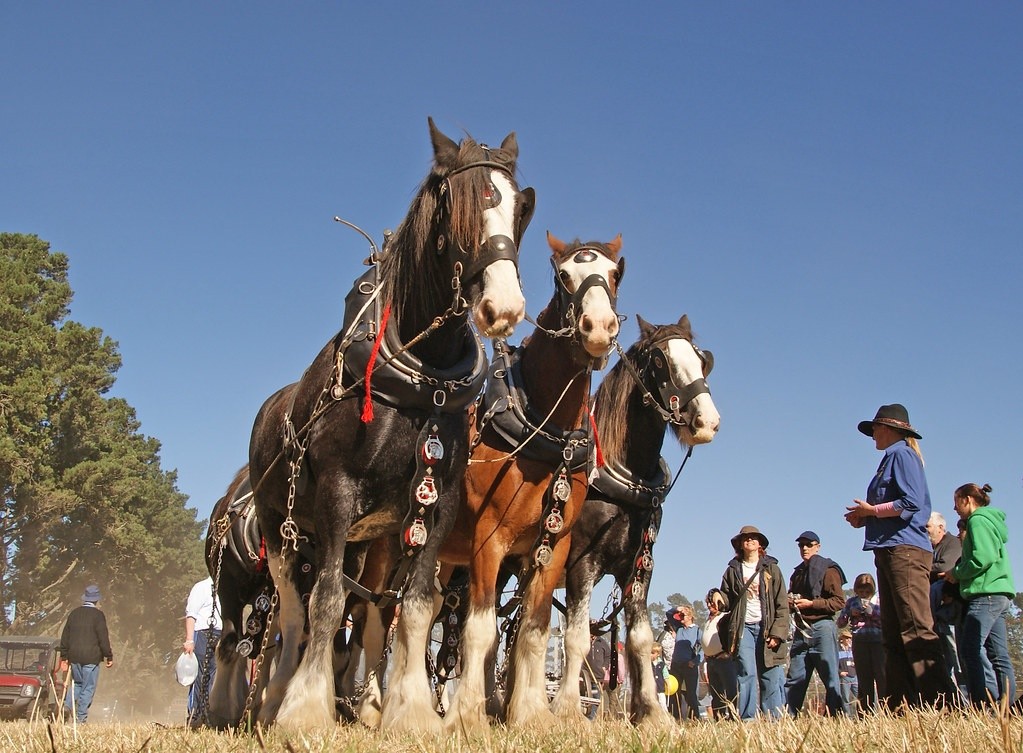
[186, 115, 725, 733]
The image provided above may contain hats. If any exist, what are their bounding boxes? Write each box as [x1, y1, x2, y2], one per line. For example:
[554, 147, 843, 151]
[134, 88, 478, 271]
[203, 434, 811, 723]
[731, 526, 769, 552]
[81, 585, 103, 601]
[857, 404, 922, 439]
[795, 530, 820, 544]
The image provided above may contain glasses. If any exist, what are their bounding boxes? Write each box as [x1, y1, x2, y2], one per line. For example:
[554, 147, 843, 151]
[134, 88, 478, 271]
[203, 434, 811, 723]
[798, 542, 818, 548]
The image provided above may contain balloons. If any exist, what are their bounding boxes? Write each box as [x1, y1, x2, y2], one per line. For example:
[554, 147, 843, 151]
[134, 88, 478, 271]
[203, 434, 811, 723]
[663, 674, 678, 695]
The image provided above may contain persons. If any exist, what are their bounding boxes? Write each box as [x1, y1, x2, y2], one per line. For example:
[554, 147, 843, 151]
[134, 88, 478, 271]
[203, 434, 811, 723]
[651, 594, 738, 723]
[838, 630, 858, 720]
[708, 526, 790, 723]
[784, 531, 848, 721]
[604, 642, 625, 720]
[183, 576, 223, 729]
[579, 619, 610, 721]
[836, 574, 887, 722]
[925, 483, 1017, 717]
[60, 585, 114, 725]
[844, 403, 964, 719]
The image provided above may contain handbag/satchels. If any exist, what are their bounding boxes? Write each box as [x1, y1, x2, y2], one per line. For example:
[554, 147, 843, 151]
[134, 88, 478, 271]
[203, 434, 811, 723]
[716, 613, 732, 653]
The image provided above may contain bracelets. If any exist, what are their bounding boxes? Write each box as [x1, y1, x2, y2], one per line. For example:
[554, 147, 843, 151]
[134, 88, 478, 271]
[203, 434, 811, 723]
[183, 639, 195, 646]
